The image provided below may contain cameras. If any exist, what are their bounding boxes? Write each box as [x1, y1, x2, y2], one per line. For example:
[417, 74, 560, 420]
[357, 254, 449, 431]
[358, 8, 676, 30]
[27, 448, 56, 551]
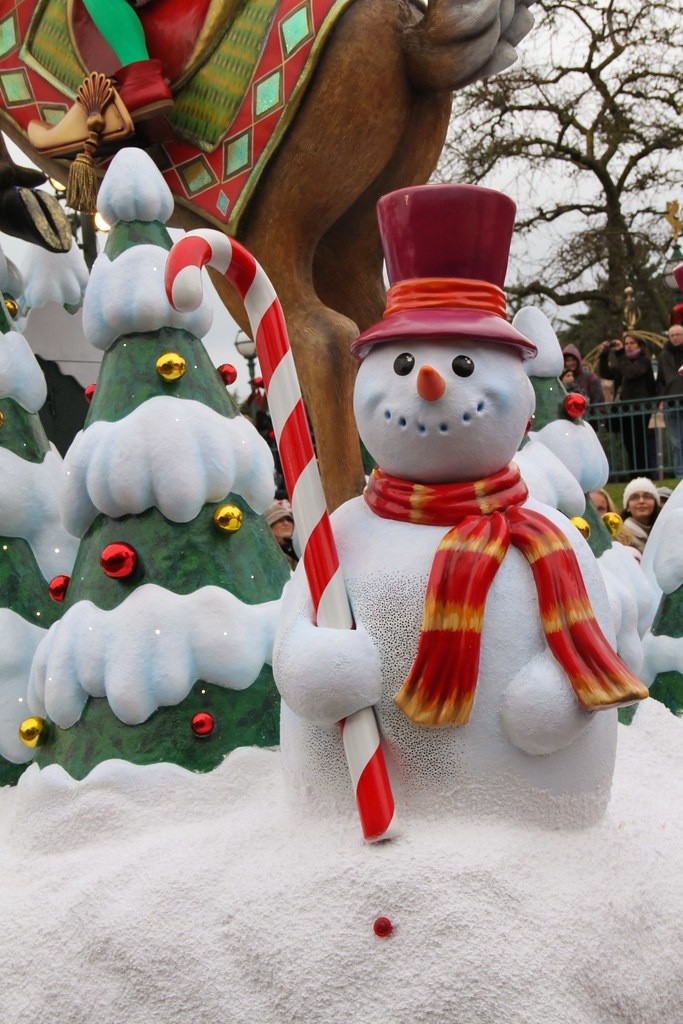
[608, 341, 616, 347]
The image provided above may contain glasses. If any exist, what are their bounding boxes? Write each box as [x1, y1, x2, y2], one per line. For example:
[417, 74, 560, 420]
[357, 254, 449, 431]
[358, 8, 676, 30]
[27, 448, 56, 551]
[630, 492, 654, 500]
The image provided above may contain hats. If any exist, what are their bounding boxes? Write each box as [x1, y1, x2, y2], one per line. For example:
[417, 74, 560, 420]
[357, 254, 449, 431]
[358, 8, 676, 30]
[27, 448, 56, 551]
[622, 477, 661, 509]
[657, 486, 673, 498]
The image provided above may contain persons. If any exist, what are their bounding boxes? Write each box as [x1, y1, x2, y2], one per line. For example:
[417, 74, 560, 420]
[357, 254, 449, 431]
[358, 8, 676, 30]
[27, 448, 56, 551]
[262, 504, 299, 572]
[586, 477, 674, 565]
[558, 324, 683, 479]
